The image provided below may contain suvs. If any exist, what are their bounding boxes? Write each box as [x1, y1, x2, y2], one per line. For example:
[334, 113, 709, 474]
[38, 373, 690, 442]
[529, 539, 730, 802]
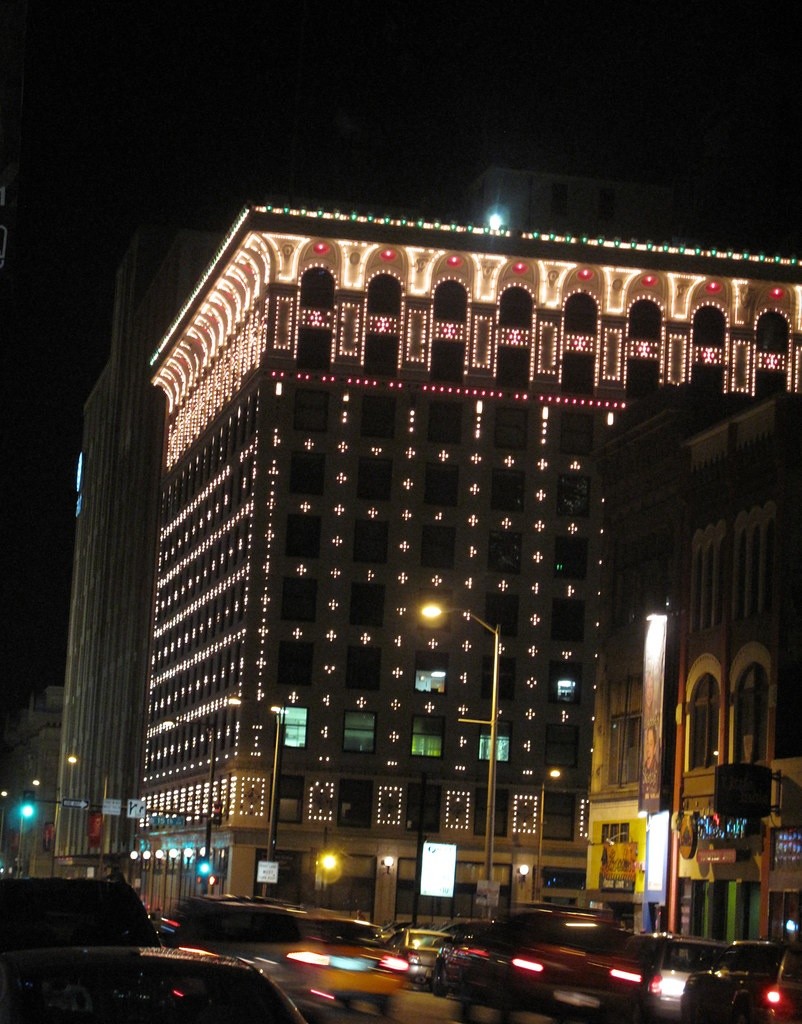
[0, 872, 407, 1024]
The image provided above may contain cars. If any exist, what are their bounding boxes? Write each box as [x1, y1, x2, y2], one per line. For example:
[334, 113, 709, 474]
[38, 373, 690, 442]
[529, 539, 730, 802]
[680, 938, 802, 1023]
[384, 929, 455, 980]
[431, 905, 657, 1023]
[643, 932, 735, 1024]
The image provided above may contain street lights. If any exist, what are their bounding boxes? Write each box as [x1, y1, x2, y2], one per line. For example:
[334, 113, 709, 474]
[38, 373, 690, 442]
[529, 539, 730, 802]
[420, 602, 501, 922]
[31, 779, 61, 877]
[67, 754, 125, 858]
[2, 791, 24, 878]
[161, 716, 221, 895]
[226, 695, 282, 897]
[532, 770, 561, 904]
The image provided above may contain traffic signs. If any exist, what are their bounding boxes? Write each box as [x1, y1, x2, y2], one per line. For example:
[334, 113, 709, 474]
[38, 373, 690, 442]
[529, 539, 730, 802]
[64, 798, 90, 810]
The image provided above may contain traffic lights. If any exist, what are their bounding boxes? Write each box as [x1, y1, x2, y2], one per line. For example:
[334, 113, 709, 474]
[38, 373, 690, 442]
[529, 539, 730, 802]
[21, 791, 35, 825]
[212, 800, 223, 826]
